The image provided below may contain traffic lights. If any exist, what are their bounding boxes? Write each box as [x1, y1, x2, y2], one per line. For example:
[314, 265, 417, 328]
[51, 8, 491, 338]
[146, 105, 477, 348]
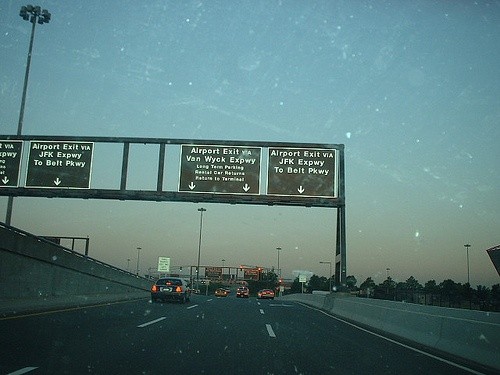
[258, 268, 261, 271]
[240, 266, 243, 269]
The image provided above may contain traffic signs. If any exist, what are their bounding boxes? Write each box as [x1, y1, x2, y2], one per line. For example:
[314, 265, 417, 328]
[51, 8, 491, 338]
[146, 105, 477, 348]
[178, 144, 262, 195]
[25, 141, 94, 189]
[265, 147, 337, 198]
[0, 140, 23, 188]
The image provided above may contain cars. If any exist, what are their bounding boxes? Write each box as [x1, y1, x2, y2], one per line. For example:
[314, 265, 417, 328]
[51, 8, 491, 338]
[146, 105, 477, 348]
[215, 288, 227, 297]
[257, 289, 275, 300]
[150, 277, 190, 303]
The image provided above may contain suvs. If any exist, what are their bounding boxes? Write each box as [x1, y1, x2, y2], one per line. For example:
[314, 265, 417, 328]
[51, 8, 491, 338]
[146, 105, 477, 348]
[236, 287, 250, 297]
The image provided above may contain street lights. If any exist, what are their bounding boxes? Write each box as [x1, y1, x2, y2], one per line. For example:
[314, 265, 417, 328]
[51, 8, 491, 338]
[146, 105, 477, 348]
[464, 244, 471, 283]
[276, 247, 282, 278]
[17, 4, 52, 135]
[196, 207, 207, 291]
[137, 248, 141, 275]
